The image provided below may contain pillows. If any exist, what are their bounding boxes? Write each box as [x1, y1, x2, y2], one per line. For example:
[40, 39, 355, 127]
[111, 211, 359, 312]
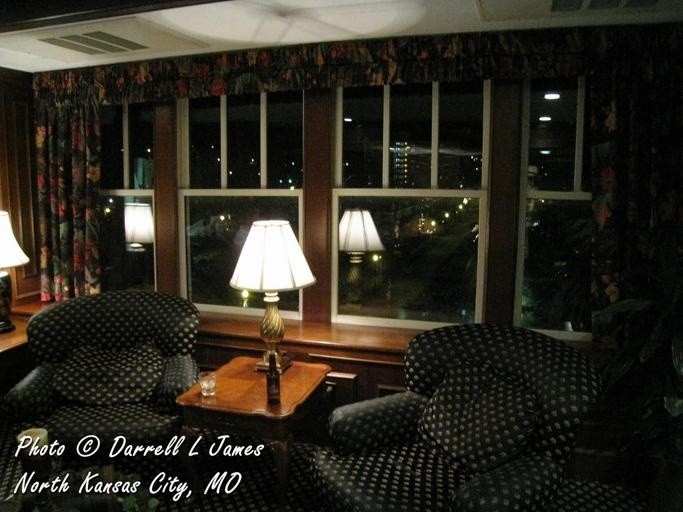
[419, 361, 542, 474]
[48, 342, 164, 406]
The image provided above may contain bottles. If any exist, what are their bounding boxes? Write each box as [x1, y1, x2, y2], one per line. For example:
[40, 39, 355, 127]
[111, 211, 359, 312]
[267, 353, 281, 405]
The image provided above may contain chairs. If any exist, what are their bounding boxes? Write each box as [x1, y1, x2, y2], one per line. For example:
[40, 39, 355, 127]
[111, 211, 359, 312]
[305, 322, 603, 511]
[3, 288, 199, 484]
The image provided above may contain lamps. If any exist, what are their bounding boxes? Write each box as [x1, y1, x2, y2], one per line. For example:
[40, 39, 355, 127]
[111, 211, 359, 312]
[338, 208, 386, 312]
[0, 208, 31, 334]
[228, 218, 316, 374]
[123, 202, 153, 286]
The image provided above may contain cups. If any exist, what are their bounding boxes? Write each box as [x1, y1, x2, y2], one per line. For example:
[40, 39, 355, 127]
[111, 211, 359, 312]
[198, 371, 217, 396]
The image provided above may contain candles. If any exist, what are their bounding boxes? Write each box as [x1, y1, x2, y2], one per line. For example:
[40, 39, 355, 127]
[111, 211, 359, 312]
[12, 426, 50, 479]
